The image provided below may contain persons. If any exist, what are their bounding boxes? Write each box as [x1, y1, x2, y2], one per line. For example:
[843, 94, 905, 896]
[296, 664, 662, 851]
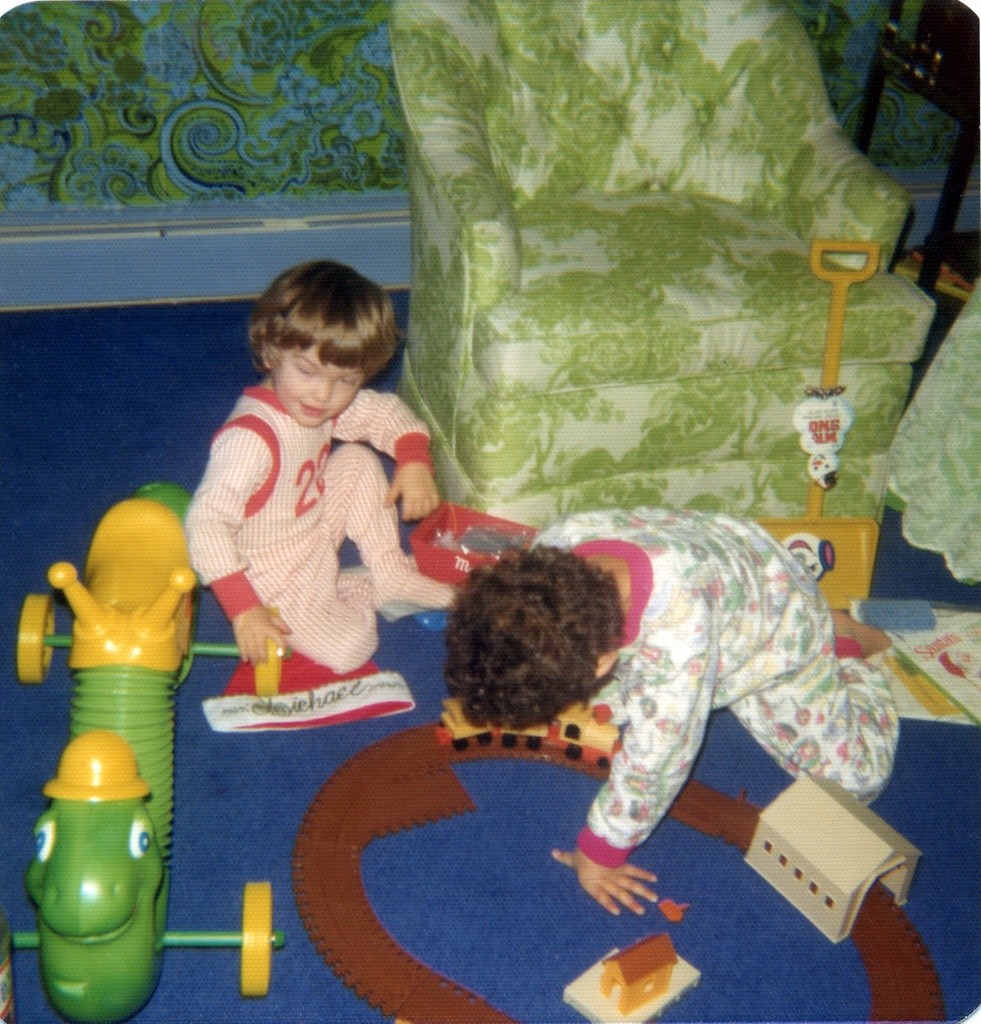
[446, 508, 900, 914]
[186, 260, 455, 674]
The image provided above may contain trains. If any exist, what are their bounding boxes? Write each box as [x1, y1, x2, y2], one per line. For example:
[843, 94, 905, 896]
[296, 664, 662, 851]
[433, 697, 620, 768]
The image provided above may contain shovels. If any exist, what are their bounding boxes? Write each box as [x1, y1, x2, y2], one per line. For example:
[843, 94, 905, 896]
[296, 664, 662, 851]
[752, 237, 884, 612]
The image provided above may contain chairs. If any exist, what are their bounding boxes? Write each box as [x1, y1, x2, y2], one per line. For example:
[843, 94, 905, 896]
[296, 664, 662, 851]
[381, 0, 938, 547]
